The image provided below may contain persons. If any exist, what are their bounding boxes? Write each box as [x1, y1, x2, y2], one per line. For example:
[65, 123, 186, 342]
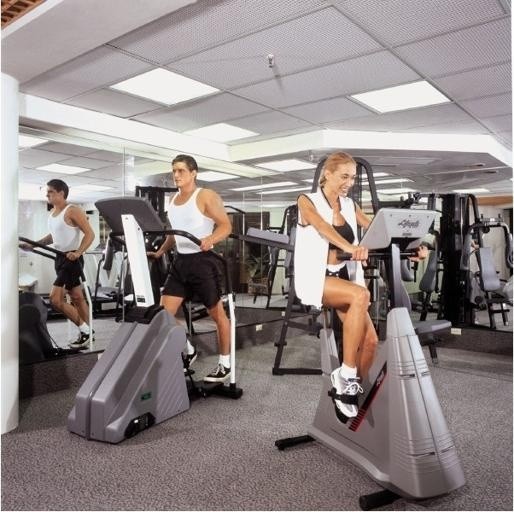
[146, 155, 231, 384]
[294, 151, 428, 418]
[19, 179, 95, 349]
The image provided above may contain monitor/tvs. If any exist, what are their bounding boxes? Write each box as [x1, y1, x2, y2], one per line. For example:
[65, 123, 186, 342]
[94, 196, 164, 233]
[358, 207, 437, 251]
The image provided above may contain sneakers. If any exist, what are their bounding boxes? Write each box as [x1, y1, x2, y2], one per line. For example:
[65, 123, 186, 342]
[204, 364, 231, 383]
[331, 367, 363, 418]
[69, 330, 95, 348]
[182, 347, 197, 372]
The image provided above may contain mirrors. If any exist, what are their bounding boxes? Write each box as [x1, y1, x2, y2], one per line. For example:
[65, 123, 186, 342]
[19, 131, 311, 364]
